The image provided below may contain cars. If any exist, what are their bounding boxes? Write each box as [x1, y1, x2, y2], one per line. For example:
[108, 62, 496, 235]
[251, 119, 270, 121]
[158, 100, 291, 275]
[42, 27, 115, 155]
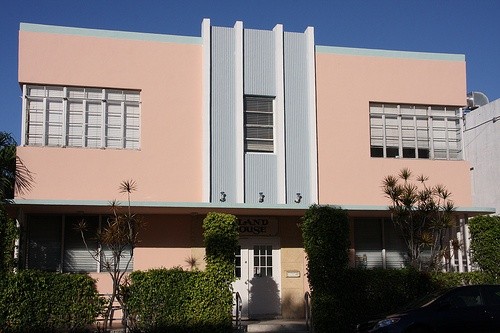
[355, 283, 500, 333]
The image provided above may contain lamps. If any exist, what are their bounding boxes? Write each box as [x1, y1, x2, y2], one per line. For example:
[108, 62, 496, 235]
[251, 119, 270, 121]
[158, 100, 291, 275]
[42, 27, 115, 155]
[258, 192, 266, 205]
[294, 192, 302, 204]
[219, 191, 227, 203]
